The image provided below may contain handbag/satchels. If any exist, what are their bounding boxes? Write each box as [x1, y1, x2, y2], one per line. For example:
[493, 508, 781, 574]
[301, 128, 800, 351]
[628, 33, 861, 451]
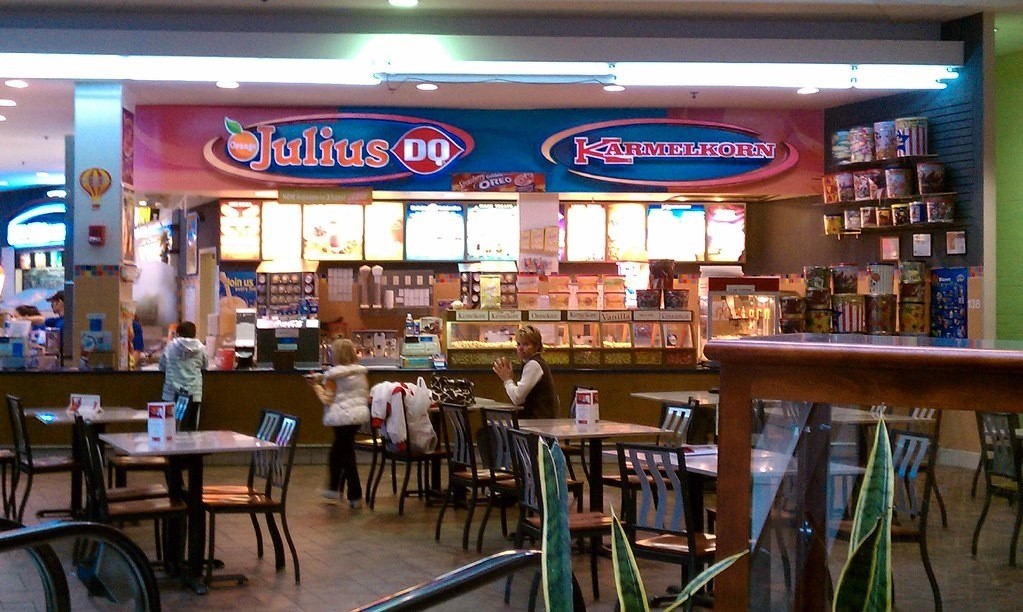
[399, 376, 438, 456]
[431, 372, 477, 408]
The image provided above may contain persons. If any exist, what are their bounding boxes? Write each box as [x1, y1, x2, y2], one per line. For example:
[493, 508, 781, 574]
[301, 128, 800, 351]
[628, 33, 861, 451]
[307, 339, 371, 508]
[159, 321, 208, 432]
[14, 290, 64, 353]
[476, 326, 559, 419]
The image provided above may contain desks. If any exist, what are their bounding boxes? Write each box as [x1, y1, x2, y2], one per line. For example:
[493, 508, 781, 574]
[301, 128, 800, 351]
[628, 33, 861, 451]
[507, 421, 672, 561]
[98, 429, 278, 590]
[24, 407, 147, 517]
[353, 397, 519, 510]
[630, 392, 780, 491]
[601, 443, 872, 612]
[756, 409, 932, 539]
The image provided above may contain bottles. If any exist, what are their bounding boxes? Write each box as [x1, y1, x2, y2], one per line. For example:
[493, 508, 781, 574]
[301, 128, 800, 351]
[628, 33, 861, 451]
[406, 313, 414, 336]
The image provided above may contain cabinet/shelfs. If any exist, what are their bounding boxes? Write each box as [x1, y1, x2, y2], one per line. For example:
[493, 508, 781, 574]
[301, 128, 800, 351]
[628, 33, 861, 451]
[825, 153, 957, 237]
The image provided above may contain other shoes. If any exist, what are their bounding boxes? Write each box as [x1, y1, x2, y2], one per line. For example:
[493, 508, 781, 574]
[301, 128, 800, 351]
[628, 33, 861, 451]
[318, 489, 344, 501]
[348, 500, 362, 512]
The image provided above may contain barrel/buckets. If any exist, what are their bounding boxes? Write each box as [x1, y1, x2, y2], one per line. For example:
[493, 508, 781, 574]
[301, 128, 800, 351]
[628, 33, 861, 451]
[779, 116, 953, 333]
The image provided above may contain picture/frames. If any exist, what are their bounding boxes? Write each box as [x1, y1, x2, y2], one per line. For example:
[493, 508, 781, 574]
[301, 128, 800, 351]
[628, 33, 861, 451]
[184, 211, 198, 274]
[912, 232, 932, 257]
[880, 236, 901, 261]
[947, 230, 967, 255]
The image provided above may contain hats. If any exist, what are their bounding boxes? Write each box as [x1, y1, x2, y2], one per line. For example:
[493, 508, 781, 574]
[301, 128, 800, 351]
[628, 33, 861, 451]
[45, 290, 64, 302]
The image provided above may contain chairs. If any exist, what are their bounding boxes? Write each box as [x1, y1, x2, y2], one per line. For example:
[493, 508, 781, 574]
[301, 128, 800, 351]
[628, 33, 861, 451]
[1, 389, 1023, 612]
